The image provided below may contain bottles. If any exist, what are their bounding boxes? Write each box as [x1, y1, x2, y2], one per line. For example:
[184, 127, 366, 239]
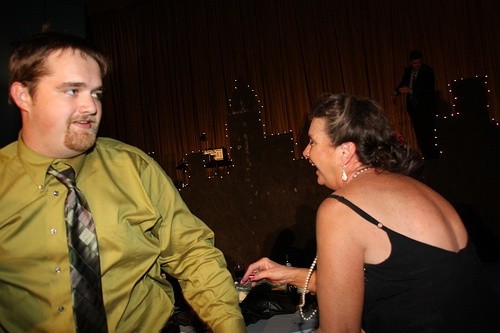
[285, 254, 298, 293]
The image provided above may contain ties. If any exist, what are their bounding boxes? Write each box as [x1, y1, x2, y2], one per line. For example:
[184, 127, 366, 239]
[47, 166, 108, 333]
[412, 72, 415, 88]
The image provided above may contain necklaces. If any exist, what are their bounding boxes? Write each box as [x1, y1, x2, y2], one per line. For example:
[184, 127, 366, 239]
[298, 166, 374, 321]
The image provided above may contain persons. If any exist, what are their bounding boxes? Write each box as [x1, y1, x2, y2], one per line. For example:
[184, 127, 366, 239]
[393, 52, 441, 161]
[240, 92, 500, 333]
[0, 32, 245, 333]
